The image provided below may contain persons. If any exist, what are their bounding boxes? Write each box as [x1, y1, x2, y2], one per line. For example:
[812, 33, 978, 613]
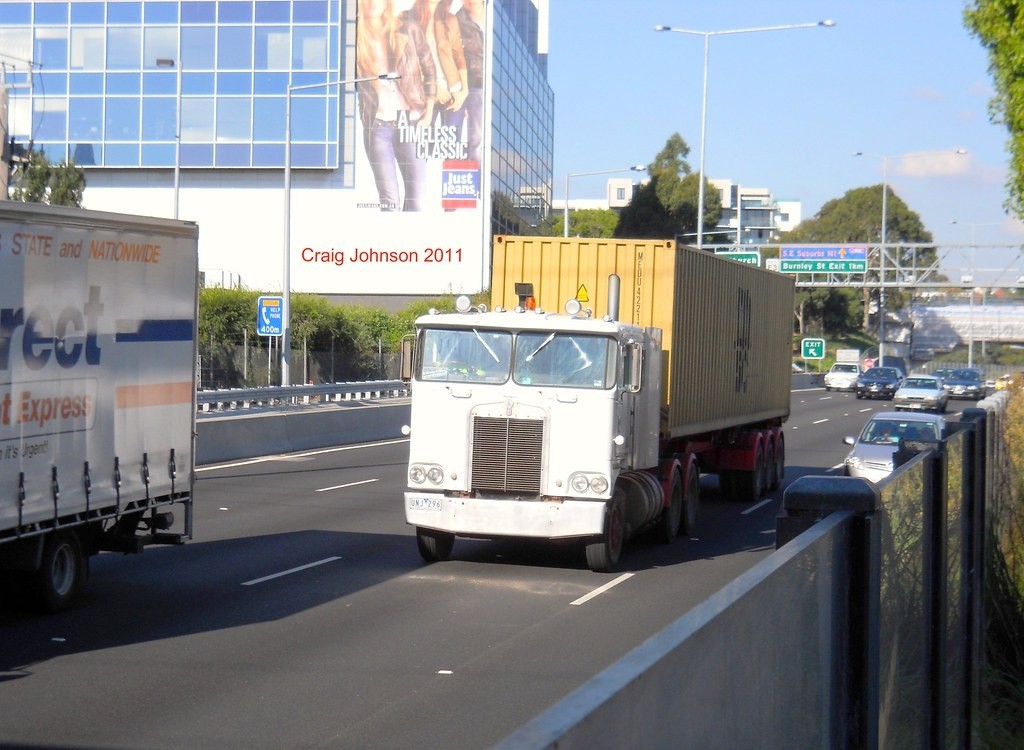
[871, 423, 899, 442]
[355, 1, 438, 211]
[399, 1, 469, 212]
[456, 0, 484, 199]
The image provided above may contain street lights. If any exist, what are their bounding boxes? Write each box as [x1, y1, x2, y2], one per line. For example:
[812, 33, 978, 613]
[563, 166, 647, 238]
[656, 20, 840, 253]
[950, 221, 1024, 368]
[852, 150, 967, 366]
[282, 72, 402, 389]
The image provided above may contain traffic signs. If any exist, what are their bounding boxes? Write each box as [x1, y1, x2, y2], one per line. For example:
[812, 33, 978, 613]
[801, 338, 825, 359]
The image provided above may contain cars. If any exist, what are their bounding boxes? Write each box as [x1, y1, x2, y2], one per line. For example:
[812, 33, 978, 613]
[825, 363, 986, 412]
[842, 411, 947, 483]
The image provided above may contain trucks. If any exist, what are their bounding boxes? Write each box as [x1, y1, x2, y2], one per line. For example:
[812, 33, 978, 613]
[0, 200, 200, 614]
[402, 234, 794, 574]
